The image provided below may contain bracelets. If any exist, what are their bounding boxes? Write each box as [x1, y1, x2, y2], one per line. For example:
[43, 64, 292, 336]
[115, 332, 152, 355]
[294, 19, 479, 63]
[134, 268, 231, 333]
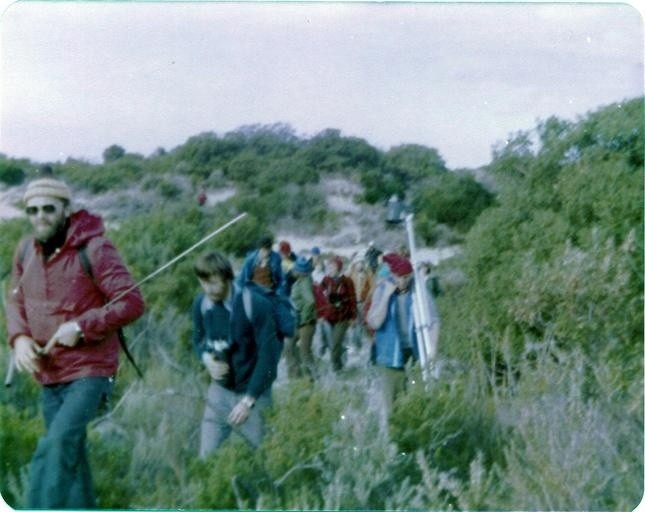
[242, 397, 255, 409]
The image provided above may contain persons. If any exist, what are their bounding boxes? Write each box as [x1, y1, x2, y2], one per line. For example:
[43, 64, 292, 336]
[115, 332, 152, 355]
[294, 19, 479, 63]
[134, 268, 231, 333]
[240, 238, 438, 372]
[4, 178, 145, 508]
[190, 253, 282, 459]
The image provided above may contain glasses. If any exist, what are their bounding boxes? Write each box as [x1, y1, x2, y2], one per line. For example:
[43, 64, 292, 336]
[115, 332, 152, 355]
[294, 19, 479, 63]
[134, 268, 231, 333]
[26, 205, 54, 213]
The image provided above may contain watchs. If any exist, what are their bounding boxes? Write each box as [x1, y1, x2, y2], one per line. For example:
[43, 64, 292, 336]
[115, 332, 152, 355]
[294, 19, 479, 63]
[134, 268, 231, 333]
[73, 320, 84, 339]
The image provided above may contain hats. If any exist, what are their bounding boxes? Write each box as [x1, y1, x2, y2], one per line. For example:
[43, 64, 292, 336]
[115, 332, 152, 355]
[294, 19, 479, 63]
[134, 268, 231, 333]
[24, 178, 71, 204]
[280, 241, 290, 253]
[294, 257, 313, 273]
[384, 254, 412, 275]
